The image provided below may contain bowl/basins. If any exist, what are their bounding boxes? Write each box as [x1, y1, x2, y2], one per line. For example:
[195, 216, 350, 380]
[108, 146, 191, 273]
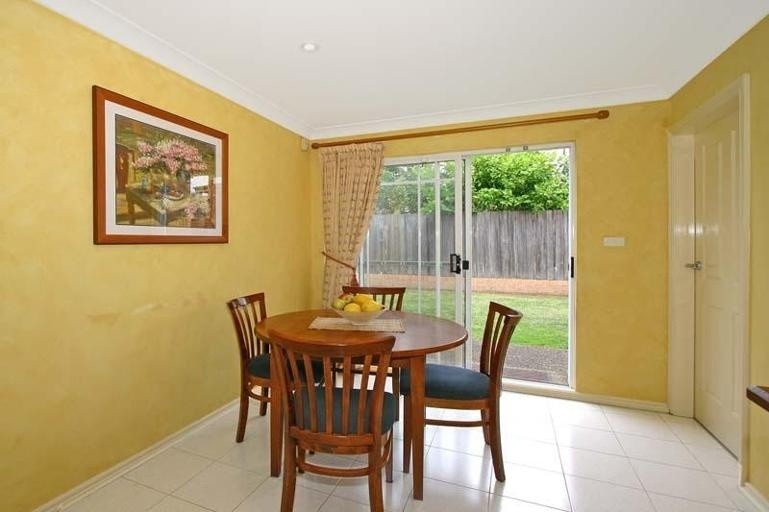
[329, 305, 388, 326]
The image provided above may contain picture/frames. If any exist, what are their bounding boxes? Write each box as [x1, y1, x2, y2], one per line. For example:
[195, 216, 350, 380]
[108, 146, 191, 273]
[91, 83, 230, 247]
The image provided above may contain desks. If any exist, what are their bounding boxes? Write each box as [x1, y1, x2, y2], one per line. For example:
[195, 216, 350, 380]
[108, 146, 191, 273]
[125, 182, 196, 226]
[254, 308, 469, 500]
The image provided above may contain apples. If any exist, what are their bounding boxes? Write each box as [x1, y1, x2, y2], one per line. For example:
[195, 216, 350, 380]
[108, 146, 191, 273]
[331, 298, 346, 309]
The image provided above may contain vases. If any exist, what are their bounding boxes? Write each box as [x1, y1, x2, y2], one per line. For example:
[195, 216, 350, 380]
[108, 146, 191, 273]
[149, 166, 190, 200]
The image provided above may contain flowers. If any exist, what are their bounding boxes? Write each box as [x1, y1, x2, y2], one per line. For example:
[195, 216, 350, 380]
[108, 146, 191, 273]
[130, 136, 208, 175]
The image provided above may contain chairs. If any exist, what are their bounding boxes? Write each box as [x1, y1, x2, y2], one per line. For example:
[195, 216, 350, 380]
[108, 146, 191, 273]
[191, 175, 215, 229]
[226, 291, 326, 445]
[115, 144, 136, 222]
[398, 299, 523, 483]
[341, 285, 406, 425]
[267, 329, 398, 512]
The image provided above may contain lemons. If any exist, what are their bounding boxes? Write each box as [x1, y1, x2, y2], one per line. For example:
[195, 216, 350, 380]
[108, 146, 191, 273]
[344, 293, 381, 312]
